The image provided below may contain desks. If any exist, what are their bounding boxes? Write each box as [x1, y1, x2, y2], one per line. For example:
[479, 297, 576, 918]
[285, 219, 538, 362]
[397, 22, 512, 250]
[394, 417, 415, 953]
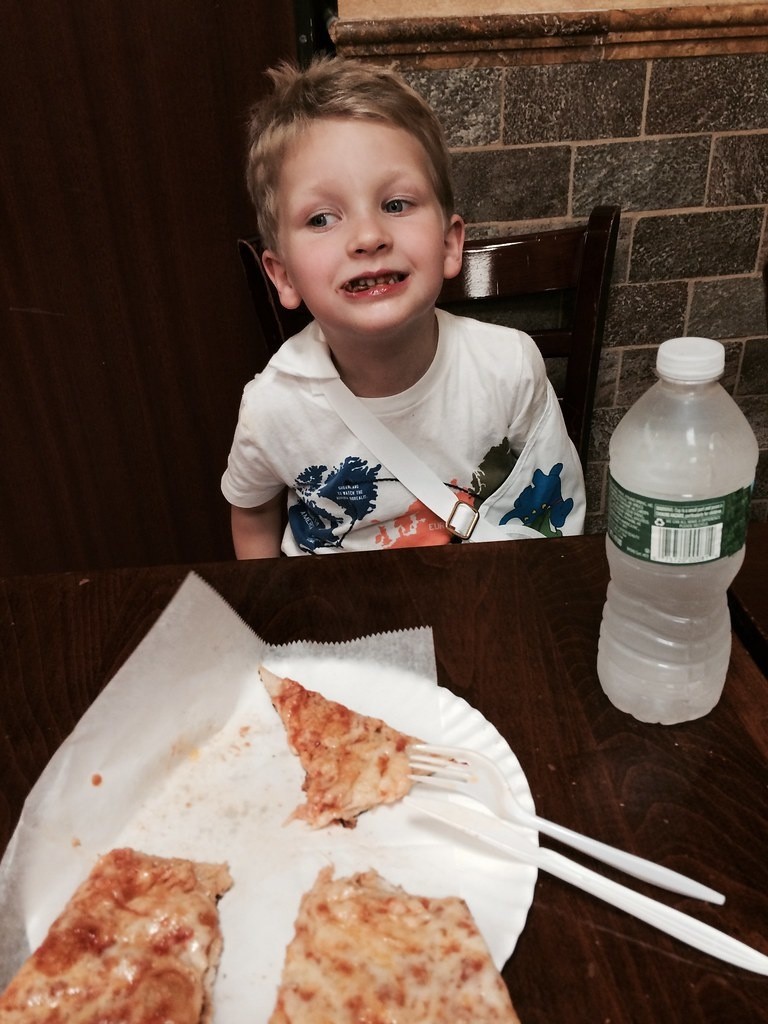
[0, 512, 768, 1024]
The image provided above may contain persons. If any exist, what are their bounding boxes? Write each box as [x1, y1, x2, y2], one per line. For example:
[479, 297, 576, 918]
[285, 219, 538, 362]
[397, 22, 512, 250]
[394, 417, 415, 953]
[221, 51, 586, 559]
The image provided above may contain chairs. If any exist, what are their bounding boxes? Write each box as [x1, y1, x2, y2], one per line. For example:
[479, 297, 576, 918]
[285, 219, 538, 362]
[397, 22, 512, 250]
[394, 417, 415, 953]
[236, 199, 624, 472]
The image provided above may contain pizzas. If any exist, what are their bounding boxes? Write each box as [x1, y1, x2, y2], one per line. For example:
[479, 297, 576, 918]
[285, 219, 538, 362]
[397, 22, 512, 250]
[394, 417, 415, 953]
[266, 865, 522, 1024]
[0, 846, 234, 1024]
[257, 666, 461, 830]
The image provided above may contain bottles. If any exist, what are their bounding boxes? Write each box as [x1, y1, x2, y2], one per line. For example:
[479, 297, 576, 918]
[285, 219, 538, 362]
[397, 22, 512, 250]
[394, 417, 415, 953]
[596, 336, 759, 727]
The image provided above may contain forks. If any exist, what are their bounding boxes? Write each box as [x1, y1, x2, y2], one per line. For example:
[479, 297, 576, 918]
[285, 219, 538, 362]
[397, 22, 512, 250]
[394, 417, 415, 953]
[407, 742, 727, 906]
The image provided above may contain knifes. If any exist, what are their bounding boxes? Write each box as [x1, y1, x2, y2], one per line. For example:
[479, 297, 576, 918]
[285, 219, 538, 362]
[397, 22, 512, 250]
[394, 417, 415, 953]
[402, 795, 768, 977]
